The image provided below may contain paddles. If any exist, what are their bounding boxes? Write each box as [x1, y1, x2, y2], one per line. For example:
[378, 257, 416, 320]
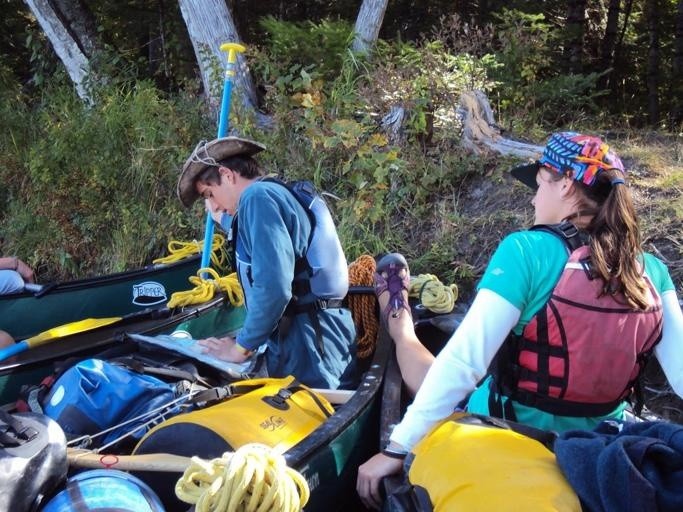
[0, 317, 122, 360]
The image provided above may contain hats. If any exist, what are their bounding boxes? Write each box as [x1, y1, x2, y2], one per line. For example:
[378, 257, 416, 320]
[510, 131, 626, 196]
[177, 136, 266, 210]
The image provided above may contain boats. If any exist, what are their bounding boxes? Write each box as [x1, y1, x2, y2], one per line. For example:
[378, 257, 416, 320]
[0, 232, 235, 342]
[380, 282, 683, 512]
[0, 283, 391, 512]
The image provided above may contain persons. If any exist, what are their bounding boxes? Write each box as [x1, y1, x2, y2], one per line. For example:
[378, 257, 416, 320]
[354, 129, 683, 512]
[0, 329, 16, 367]
[174, 135, 358, 411]
[0, 256, 39, 296]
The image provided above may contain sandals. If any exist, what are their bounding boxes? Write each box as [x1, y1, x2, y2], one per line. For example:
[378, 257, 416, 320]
[372, 252, 411, 334]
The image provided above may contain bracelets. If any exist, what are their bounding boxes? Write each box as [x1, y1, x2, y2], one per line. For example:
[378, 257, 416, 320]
[379, 448, 407, 460]
[234, 344, 252, 356]
[13, 256, 18, 270]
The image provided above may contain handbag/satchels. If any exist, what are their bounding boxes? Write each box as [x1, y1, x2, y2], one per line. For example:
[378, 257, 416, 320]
[32, 355, 196, 457]
[128, 374, 337, 512]
